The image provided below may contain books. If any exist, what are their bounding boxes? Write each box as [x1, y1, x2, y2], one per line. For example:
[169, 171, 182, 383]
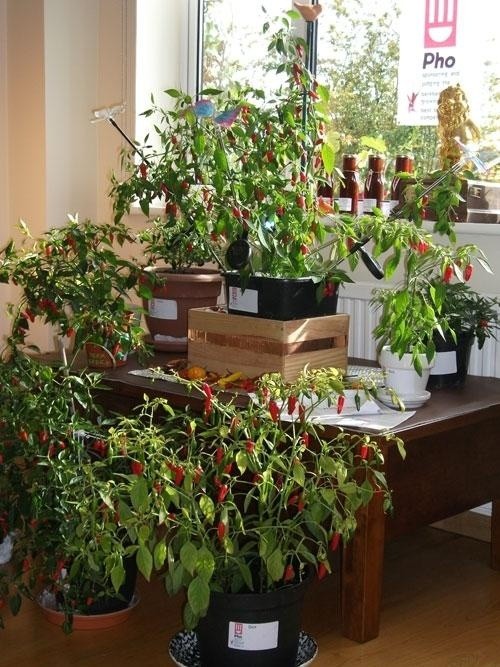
[247, 386, 397, 422]
[241, 408, 418, 435]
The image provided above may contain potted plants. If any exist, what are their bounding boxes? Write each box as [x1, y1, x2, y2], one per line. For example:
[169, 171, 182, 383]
[0, 3, 499, 667]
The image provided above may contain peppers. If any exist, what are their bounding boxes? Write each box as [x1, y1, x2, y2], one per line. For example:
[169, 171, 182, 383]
[0, 44, 472, 605]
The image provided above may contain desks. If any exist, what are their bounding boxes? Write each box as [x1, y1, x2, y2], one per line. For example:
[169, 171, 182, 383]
[25, 333, 499, 644]
